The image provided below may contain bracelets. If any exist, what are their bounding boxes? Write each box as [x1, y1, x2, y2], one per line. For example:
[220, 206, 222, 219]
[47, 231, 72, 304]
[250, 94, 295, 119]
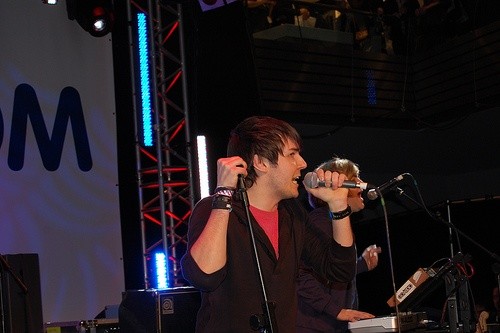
[211, 194, 232, 209]
[214, 186, 235, 195]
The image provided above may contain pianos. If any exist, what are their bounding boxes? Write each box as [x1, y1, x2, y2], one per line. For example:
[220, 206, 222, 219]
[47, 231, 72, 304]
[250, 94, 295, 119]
[348, 268, 464, 333]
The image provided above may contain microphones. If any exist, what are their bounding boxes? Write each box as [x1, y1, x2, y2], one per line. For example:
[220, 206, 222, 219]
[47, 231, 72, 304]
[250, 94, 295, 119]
[367, 174, 403, 200]
[303, 172, 358, 189]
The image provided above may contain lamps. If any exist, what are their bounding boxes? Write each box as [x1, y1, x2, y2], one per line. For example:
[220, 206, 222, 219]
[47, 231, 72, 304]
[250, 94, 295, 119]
[66, 0, 115, 37]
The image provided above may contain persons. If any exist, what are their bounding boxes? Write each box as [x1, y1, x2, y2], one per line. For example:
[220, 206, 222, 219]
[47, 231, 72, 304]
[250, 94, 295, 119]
[179, 115, 376, 333]
[478, 283, 500, 333]
[293, 8, 317, 31]
[296, 157, 381, 333]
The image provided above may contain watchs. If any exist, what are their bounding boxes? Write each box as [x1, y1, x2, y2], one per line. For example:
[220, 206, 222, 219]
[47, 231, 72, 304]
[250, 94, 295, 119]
[329, 205, 352, 220]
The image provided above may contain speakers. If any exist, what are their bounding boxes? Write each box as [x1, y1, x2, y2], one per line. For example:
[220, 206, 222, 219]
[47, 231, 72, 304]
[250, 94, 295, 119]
[0, 253, 43, 333]
